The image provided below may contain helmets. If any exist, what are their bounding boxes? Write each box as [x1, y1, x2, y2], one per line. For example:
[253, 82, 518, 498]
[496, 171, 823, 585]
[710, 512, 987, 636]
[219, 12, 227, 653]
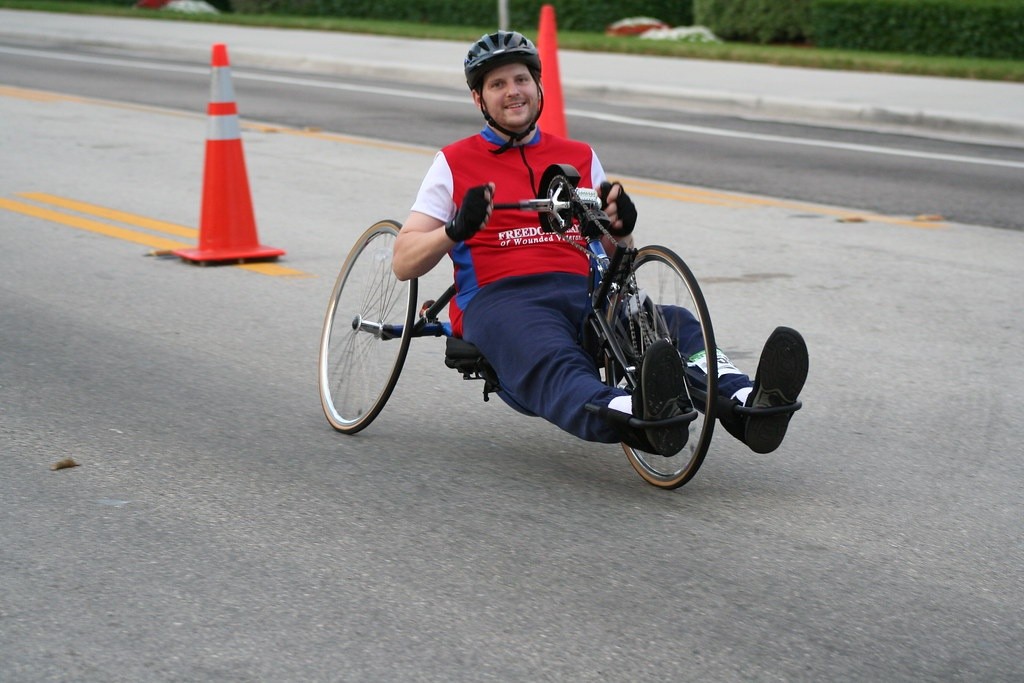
[464, 29, 541, 90]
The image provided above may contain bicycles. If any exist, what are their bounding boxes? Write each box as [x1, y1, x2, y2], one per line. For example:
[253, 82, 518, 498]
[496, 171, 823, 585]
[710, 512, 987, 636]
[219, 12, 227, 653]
[317, 163, 803, 491]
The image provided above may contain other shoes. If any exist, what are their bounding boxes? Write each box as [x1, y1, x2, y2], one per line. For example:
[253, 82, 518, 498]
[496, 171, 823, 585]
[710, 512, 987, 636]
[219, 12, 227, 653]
[739, 326, 809, 454]
[632, 340, 689, 456]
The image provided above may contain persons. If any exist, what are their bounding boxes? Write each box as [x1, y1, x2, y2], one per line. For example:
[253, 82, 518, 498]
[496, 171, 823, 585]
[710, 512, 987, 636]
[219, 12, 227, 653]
[391, 28, 810, 457]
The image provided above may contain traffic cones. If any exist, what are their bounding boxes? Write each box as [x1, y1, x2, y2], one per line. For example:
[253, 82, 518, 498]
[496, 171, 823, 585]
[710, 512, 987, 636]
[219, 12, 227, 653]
[168, 44, 286, 265]
[532, 4, 569, 136]
